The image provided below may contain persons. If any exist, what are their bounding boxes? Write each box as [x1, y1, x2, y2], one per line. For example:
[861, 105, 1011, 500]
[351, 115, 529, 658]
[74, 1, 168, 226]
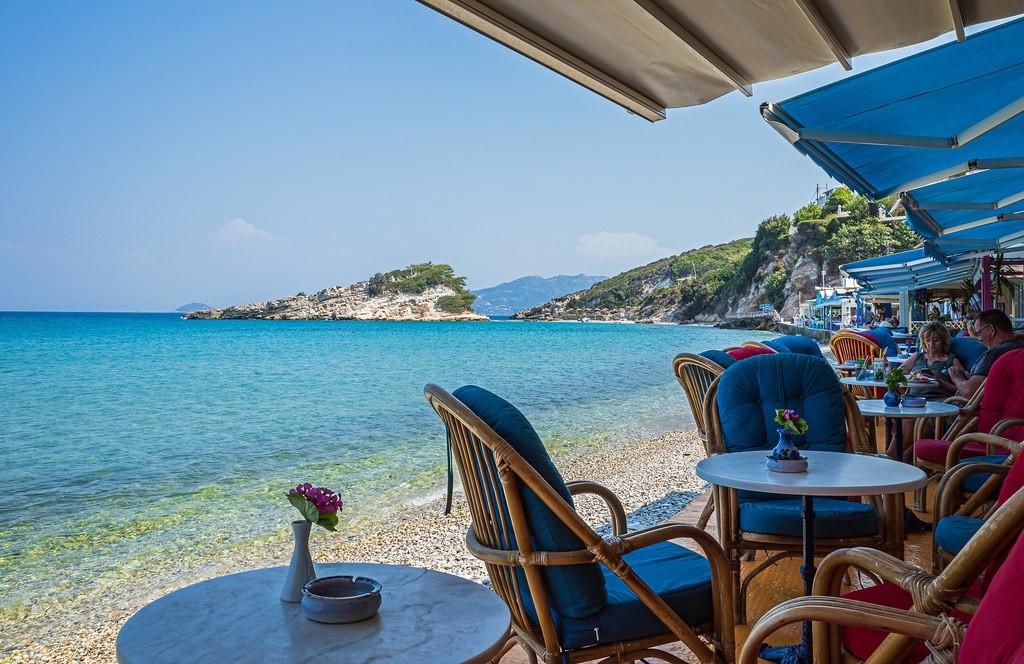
[932, 308, 1024, 436]
[866, 306, 888, 324]
[885, 321, 970, 462]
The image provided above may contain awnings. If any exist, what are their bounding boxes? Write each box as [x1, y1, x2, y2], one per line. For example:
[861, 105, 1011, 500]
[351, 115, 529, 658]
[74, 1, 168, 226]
[840, 241, 1024, 292]
[899, 166, 1024, 266]
[414, 0, 1024, 124]
[759, 17, 1024, 201]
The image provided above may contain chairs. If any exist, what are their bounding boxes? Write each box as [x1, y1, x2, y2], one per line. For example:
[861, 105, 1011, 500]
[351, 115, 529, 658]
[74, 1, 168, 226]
[424, 327, 1024, 664]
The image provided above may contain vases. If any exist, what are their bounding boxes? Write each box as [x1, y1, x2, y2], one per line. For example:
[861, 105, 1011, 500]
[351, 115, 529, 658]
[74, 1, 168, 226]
[281, 520, 318, 602]
[766, 428, 808, 473]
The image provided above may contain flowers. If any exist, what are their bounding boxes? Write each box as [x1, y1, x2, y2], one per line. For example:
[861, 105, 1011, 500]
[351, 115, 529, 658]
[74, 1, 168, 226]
[773, 410, 806, 435]
[285, 481, 344, 529]
[905, 339, 914, 346]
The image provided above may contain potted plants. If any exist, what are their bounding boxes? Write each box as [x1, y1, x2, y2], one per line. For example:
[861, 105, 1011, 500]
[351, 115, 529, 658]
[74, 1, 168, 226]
[880, 367, 907, 407]
[854, 356, 867, 380]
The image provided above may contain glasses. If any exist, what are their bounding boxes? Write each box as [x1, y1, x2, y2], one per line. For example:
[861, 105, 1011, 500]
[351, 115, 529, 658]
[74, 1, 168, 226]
[975, 325, 991, 337]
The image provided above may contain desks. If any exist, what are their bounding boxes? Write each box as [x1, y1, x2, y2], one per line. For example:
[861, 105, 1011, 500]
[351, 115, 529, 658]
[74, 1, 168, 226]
[856, 399, 959, 527]
[695, 450, 927, 661]
[887, 357, 911, 363]
[116, 562, 512, 664]
[839, 375, 940, 461]
[831, 363, 857, 393]
